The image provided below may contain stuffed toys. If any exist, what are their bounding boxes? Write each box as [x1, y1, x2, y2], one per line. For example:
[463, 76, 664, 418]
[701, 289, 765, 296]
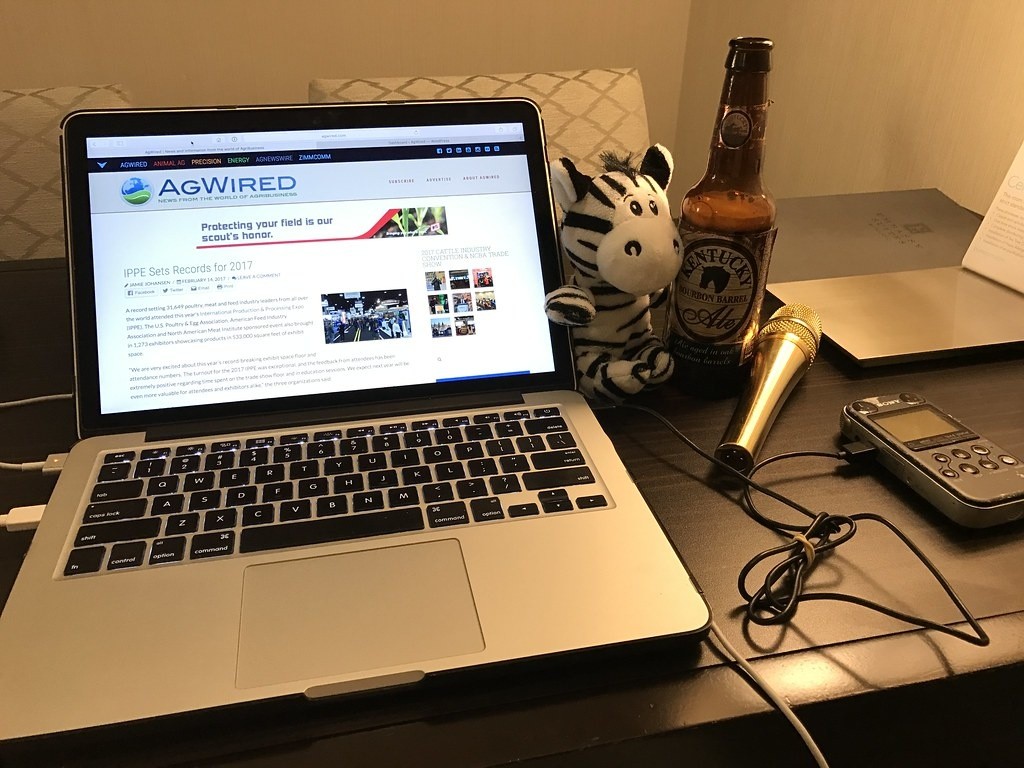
[545, 141, 684, 402]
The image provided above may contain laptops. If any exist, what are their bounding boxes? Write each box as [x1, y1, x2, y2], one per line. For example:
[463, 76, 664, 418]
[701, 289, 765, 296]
[0, 96, 714, 753]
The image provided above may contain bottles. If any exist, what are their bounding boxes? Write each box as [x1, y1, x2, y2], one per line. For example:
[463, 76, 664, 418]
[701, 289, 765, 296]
[663, 38, 778, 400]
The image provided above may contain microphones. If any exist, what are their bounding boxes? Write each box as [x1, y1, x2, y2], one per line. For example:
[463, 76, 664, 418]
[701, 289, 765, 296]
[719, 304, 822, 479]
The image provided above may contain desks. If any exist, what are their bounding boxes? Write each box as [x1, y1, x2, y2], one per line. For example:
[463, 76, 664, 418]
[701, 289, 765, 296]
[0, 189, 1024, 768]
